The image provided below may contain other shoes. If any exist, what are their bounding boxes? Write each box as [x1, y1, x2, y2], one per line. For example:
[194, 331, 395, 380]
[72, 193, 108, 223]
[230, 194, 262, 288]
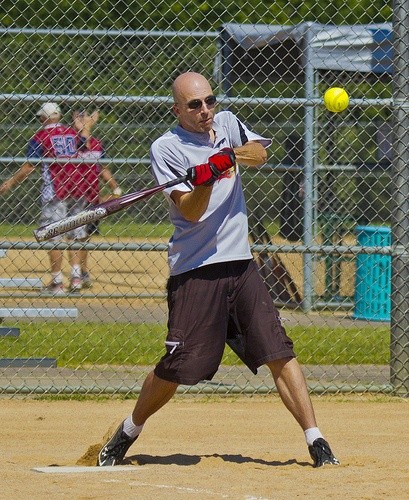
[46, 279, 63, 292]
[82, 272, 91, 289]
[69, 278, 82, 291]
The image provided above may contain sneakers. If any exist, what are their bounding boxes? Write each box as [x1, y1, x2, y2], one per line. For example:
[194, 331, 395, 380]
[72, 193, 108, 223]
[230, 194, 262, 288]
[97, 420, 140, 466]
[308, 437, 340, 468]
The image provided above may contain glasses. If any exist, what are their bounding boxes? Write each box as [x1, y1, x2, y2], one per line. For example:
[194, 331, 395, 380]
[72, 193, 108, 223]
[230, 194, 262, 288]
[177, 95, 217, 109]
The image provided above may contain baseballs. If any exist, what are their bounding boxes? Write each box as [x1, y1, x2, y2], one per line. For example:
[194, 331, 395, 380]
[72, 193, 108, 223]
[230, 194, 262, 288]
[322, 87, 351, 112]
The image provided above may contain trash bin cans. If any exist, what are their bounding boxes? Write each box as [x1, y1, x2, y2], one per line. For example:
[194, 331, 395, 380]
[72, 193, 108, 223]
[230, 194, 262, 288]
[350, 226, 392, 322]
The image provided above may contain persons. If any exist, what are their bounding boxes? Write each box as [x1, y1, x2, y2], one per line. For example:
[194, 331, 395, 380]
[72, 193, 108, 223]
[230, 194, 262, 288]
[96, 72, 340, 468]
[0, 103, 122, 294]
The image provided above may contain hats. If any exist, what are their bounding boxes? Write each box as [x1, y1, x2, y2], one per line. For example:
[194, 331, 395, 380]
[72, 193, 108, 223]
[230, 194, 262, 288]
[36, 101, 60, 118]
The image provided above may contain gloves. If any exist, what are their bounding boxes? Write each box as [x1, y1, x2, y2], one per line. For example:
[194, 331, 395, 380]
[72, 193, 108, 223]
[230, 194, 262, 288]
[187, 163, 217, 187]
[207, 147, 238, 180]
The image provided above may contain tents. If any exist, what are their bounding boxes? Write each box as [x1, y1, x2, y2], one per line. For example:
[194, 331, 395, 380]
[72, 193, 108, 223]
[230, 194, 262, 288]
[213, 21, 394, 309]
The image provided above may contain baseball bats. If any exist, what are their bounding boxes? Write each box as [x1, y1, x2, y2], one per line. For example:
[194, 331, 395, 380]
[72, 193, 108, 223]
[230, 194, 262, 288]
[33, 174, 188, 242]
